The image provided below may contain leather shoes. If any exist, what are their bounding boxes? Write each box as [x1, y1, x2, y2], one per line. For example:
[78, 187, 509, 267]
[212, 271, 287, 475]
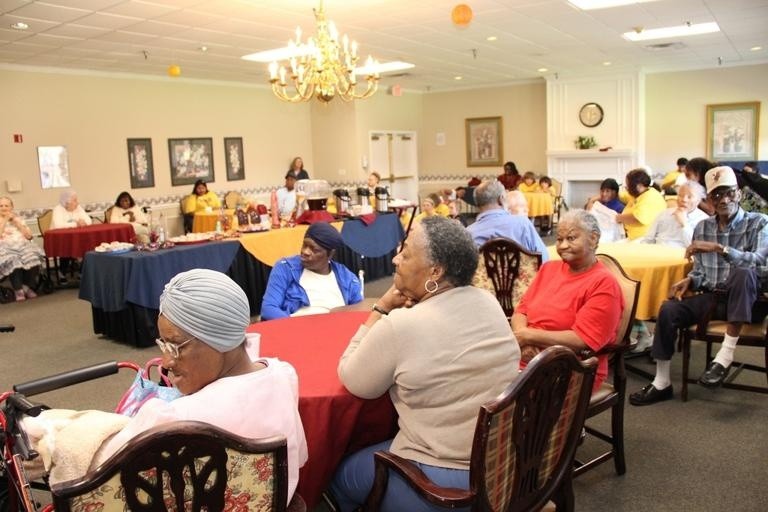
[629, 384, 673, 406]
[698, 362, 732, 388]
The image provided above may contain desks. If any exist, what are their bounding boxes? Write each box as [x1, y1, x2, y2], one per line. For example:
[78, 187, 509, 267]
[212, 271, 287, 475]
[42, 224, 133, 285]
[546, 240, 692, 350]
[85, 236, 237, 343]
[342, 208, 398, 284]
[209, 220, 343, 318]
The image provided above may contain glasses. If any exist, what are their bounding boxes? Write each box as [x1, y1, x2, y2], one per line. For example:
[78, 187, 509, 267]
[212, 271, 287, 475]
[155, 338, 194, 358]
[710, 188, 736, 200]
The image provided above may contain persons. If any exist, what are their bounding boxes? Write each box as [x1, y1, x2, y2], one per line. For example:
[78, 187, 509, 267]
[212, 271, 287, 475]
[413, 198, 439, 230]
[434, 186, 467, 205]
[103, 269, 308, 508]
[328, 217, 521, 512]
[367, 173, 385, 195]
[0, 198, 42, 302]
[518, 172, 538, 224]
[537, 176, 556, 235]
[50, 190, 92, 285]
[447, 200, 467, 227]
[661, 157, 688, 194]
[685, 157, 768, 215]
[110, 191, 150, 244]
[743, 161, 761, 177]
[275, 172, 309, 221]
[621, 181, 709, 353]
[288, 157, 309, 180]
[184, 180, 222, 233]
[496, 162, 521, 191]
[465, 181, 549, 266]
[628, 166, 768, 406]
[260, 221, 362, 321]
[511, 210, 624, 395]
[584, 179, 625, 214]
[585, 169, 667, 245]
[425, 193, 449, 219]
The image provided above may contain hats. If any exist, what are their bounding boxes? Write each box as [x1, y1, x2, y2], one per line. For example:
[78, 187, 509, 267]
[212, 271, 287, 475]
[704, 166, 737, 194]
[158, 269, 250, 351]
[285, 172, 296, 179]
[304, 221, 341, 251]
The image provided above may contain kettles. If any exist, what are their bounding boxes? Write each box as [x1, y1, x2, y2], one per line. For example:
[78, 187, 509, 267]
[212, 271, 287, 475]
[375, 185, 390, 214]
[357, 188, 371, 206]
[332, 189, 352, 215]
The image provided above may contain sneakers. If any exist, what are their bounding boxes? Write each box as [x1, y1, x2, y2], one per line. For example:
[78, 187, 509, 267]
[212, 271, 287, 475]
[14, 289, 25, 301]
[22, 284, 37, 298]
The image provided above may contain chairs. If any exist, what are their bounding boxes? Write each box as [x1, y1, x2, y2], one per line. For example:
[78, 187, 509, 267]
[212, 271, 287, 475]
[523, 191, 550, 230]
[317, 344, 598, 512]
[545, 178, 562, 227]
[37, 208, 77, 282]
[180, 194, 192, 237]
[49, 421, 288, 512]
[225, 190, 246, 211]
[563, 254, 641, 480]
[469, 238, 543, 326]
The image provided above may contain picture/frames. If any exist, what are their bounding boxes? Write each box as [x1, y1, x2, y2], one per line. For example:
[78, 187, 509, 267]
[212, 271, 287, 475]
[705, 101, 761, 165]
[167, 136, 215, 187]
[223, 135, 245, 181]
[126, 137, 155, 189]
[465, 115, 504, 167]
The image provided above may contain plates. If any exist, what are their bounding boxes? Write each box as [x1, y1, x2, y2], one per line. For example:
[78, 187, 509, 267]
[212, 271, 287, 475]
[238, 229, 269, 233]
[95, 245, 133, 256]
[174, 238, 210, 245]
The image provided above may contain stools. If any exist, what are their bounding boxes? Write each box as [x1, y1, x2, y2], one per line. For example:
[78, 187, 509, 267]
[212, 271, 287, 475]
[678, 298, 768, 401]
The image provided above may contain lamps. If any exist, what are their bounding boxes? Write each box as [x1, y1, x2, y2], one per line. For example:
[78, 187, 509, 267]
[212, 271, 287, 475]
[268, 0, 380, 105]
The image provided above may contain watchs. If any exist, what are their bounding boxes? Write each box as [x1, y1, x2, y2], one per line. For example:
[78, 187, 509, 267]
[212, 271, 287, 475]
[372, 305, 389, 316]
